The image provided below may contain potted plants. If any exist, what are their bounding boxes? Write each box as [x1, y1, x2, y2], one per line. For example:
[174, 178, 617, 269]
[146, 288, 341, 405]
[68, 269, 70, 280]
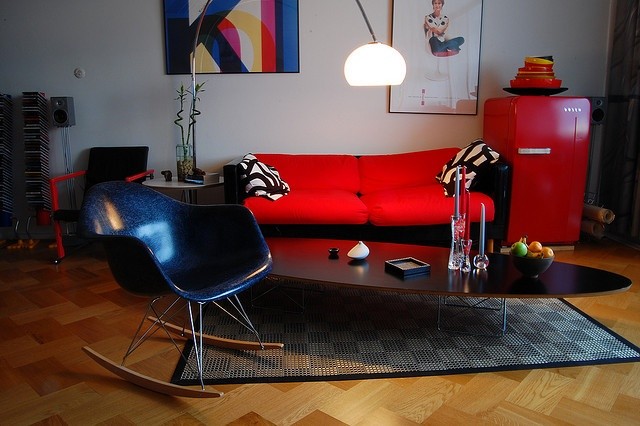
[174, 81, 206, 181]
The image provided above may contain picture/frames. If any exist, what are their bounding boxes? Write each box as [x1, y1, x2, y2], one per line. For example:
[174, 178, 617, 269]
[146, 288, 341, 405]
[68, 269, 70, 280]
[389, 0, 483, 115]
[162, 0, 300, 74]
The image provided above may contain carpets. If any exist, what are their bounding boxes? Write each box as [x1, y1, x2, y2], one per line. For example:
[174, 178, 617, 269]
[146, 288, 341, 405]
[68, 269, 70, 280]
[171, 278, 640, 386]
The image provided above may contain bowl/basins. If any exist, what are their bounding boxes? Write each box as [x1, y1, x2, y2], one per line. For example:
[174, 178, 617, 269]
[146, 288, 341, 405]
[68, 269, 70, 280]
[511, 256, 555, 279]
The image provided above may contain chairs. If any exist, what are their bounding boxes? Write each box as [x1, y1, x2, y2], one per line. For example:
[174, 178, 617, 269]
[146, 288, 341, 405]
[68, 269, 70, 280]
[49, 147, 154, 264]
[78, 181, 285, 398]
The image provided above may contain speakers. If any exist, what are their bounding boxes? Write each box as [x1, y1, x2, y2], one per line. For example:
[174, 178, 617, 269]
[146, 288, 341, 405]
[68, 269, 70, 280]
[52, 97, 76, 127]
[591, 98, 606, 123]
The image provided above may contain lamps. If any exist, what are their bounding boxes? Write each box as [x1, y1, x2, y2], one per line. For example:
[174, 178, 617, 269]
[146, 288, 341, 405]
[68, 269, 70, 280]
[343, 0, 406, 87]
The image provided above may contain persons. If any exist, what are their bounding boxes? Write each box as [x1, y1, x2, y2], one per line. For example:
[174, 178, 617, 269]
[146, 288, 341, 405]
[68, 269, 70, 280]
[424, 0, 464, 52]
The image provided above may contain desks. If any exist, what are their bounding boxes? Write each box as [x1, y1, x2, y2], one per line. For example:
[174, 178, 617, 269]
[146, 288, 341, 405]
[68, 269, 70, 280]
[237, 237, 632, 337]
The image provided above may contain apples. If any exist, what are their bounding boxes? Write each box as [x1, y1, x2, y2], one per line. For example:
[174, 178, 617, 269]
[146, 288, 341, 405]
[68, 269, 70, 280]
[540, 247, 553, 258]
[511, 242, 527, 257]
[529, 241, 542, 253]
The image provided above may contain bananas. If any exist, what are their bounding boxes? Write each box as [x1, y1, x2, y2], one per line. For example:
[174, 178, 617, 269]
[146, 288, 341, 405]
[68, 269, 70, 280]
[519, 235, 544, 258]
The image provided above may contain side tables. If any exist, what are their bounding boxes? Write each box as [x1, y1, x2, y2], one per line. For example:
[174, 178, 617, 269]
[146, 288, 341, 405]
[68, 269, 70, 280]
[143, 174, 224, 205]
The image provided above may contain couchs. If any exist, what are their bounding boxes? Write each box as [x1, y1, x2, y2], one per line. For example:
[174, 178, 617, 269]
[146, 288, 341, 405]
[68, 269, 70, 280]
[223, 147, 513, 250]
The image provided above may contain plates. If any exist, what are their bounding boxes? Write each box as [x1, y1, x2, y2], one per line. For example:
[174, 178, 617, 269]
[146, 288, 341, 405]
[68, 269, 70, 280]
[503, 88, 568, 96]
[510, 56, 562, 88]
